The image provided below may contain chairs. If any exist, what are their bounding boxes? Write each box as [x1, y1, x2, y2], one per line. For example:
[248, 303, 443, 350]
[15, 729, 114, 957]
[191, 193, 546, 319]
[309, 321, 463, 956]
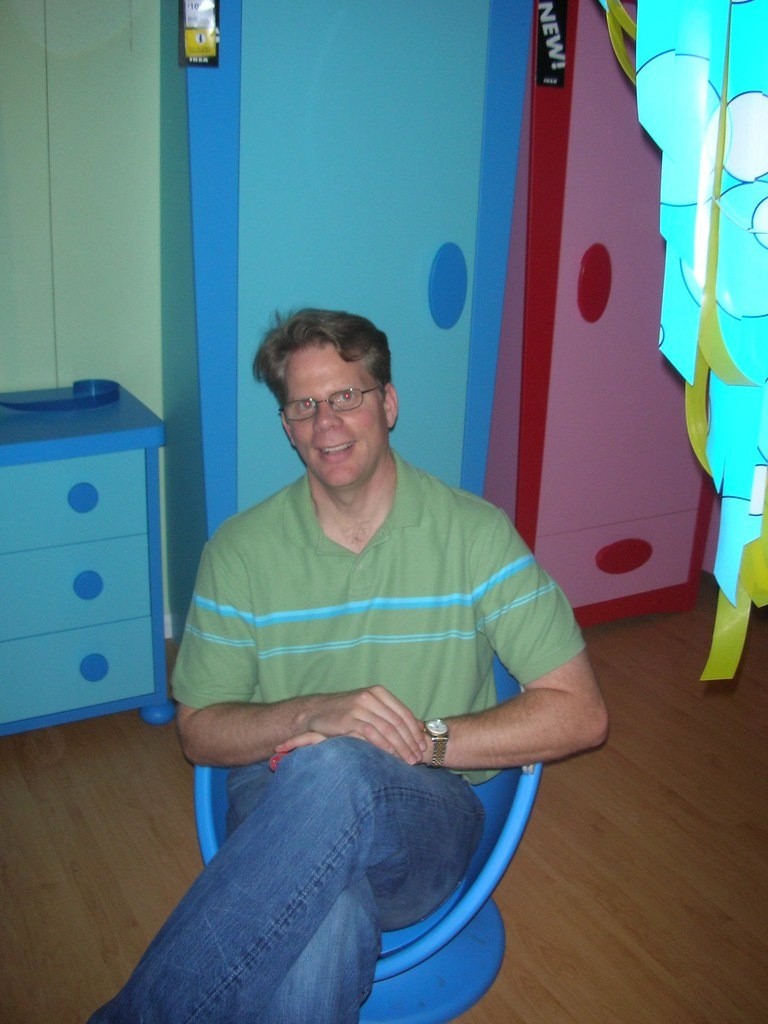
[194, 650, 544, 1024]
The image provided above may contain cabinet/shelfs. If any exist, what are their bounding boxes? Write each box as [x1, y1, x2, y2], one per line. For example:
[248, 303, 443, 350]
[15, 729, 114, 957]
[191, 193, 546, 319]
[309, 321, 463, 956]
[0, 378, 177, 732]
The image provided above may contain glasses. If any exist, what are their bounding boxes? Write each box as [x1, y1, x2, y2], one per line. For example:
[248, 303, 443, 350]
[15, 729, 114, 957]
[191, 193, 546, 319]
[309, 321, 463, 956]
[279, 384, 382, 421]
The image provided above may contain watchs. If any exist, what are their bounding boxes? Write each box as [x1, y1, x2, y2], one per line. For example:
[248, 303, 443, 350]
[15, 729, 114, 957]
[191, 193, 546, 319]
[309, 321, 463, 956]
[423, 718, 450, 768]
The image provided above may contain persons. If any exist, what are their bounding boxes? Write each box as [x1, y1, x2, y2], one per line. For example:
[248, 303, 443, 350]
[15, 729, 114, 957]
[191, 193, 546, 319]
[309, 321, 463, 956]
[82, 308, 611, 1024]
